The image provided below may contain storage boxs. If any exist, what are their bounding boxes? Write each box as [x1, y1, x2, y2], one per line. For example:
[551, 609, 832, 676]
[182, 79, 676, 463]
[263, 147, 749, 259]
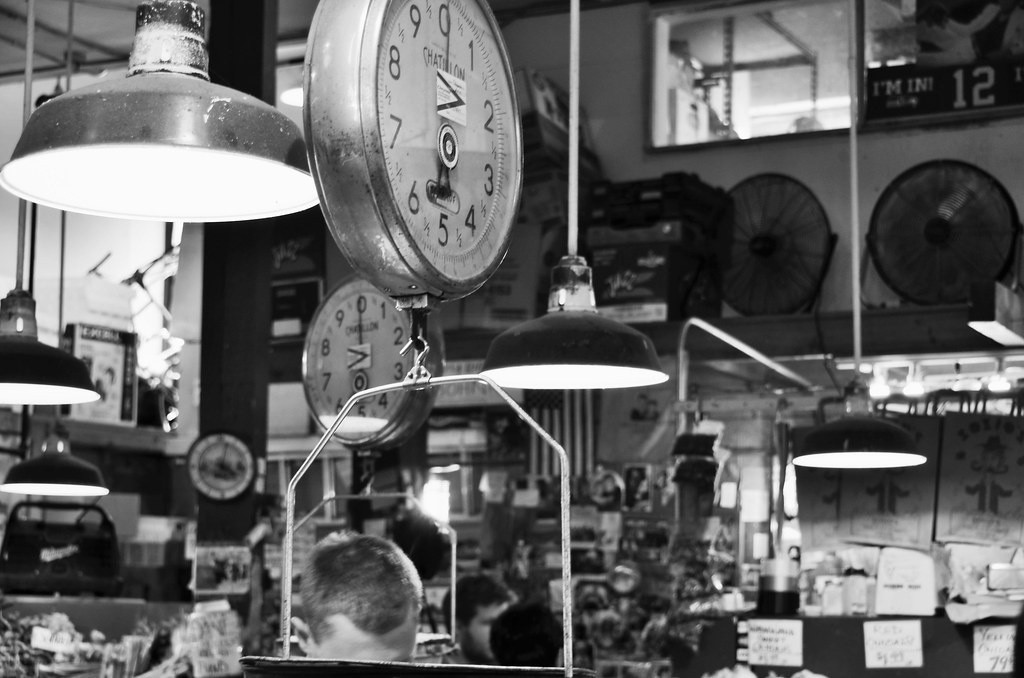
[866, 49, 1024, 119]
[62, 320, 139, 427]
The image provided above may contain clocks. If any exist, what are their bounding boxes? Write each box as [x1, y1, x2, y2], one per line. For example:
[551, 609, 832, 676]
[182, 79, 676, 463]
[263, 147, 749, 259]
[185, 433, 255, 504]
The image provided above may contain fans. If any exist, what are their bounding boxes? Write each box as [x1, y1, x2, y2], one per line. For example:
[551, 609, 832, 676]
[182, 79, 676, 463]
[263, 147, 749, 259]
[716, 173, 831, 313]
[864, 157, 1021, 306]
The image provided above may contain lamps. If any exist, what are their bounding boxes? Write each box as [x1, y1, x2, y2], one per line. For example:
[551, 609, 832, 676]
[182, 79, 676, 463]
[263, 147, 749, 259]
[0, 0, 322, 222]
[0, 0, 105, 410]
[788, 0, 933, 472]
[0, 51, 113, 500]
[478, 0, 673, 390]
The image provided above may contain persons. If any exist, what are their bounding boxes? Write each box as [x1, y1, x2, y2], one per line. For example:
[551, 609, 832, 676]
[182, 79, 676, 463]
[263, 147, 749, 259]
[291, 530, 424, 665]
[159, 466, 624, 678]
